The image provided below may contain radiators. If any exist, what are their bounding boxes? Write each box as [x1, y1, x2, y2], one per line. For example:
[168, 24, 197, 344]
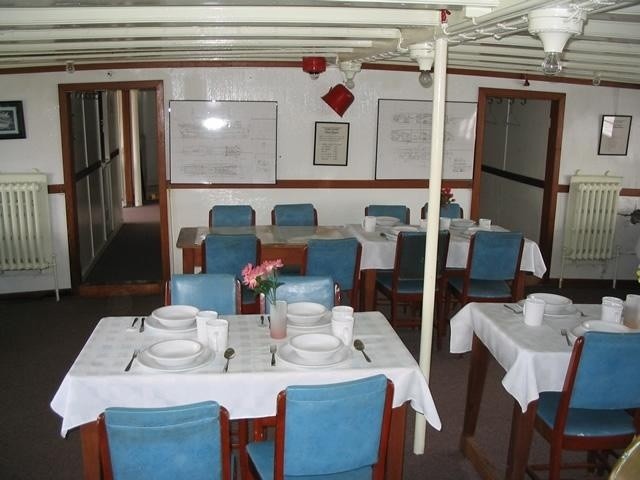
[559, 172, 625, 263]
[1, 170, 56, 273]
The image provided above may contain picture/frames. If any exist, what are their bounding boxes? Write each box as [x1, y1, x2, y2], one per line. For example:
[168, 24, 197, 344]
[311, 120, 350, 168]
[596, 113, 633, 157]
[0, 98, 27, 142]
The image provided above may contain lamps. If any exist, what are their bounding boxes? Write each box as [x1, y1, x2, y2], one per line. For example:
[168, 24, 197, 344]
[527, 7, 588, 77]
[337, 60, 364, 89]
[407, 42, 436, 88]
[301, 54, 329, 81]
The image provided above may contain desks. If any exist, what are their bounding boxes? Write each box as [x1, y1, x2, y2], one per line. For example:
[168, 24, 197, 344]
[177, 225, 535, 310]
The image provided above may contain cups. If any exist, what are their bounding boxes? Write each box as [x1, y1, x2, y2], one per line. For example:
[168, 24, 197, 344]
[333, 304, 354, 316]
[195, 310, 219, 339]
[440, 216, 451, 230]
[521, 298, 547, 327]
[361, 216, 377, 233]
[331, 316, 355, 348]
[601, 295, 624, 325]
[624, 295, 640, 330]
[478, 219, 492, 230]
[205, 319, 229, 351]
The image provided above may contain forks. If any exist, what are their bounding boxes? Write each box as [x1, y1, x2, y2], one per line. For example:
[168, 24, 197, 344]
[575, 308, 592, 318]
[269, 344, 278, 367]
[124, 349, 141, 372]
[559, 326, 573, 347]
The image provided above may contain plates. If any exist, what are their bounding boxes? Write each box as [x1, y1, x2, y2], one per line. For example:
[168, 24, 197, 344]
[518, 299, 577, 318]
[144, 314, 196, 333]
[288, 312, 331, 329]
[137, 347, 216, 373]
[384, 231, 398, 241]
[276, 343, 353, 368]
[376, 223, 400, 228]
[570, 325, 584, 343]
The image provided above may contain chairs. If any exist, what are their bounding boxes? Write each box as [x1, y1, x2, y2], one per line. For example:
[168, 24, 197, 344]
[259, 274, 341, 314]
[164, 273, 243, 316]
[524, 329, 639, 479]
[301, 237, 362, 310]
[245, 373, 398, 480]
[96, 399, 233, 479]
[375, 230, 449, 354]
[271, 204, 318, 226]
[444, 230, 525, 357]
[208, 205, 256, 228]
[420, 203, 463, 219]
[200, 233, 261, 313]
[365, 204, 410, 225]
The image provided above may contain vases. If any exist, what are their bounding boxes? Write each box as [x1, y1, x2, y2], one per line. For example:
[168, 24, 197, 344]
[269, 298, 289, 341]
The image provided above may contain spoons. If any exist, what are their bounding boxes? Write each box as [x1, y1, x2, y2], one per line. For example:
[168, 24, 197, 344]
[354, 339, 372, 363]
[222, 347, 235, 372]
[380, 233, 389, 242]
[502, 304, 522, 315]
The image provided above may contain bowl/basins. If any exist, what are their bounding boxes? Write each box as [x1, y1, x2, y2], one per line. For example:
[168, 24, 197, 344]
[152, 304, 200, 327]
[375, 216, 401, 225]
[285, 301, 330, 323]
[393, 226, 418, 234]
[146, 339, 205, 367]
[451, 218, 476, 226]
[290, 332, 344, 360]
[580, 318, 632, 340]
[528, 291, 574, 313]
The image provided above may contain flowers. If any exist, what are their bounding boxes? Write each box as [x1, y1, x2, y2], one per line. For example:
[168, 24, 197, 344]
[242, 259, 279, 305]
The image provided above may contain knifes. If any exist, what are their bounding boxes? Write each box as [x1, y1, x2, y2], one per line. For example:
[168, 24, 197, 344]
[127, 318, 137, 330]
[260, 316, 266, 327]
[140, 317, 145, 333]
